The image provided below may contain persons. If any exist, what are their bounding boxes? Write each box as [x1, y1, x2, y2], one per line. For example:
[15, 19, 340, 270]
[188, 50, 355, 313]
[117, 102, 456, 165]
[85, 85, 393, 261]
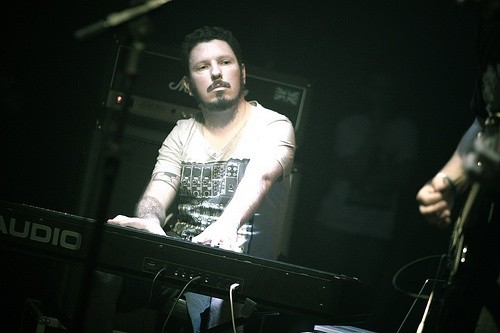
[104, 26, 298, 333]
[416, 108, 485, 232]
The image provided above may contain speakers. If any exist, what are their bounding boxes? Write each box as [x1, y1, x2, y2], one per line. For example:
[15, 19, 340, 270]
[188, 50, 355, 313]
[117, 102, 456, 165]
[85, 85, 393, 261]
[76, 40, 313, 333]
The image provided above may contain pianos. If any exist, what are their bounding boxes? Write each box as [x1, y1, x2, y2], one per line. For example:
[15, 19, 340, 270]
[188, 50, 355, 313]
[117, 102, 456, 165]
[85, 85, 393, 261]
[0, 200, 371, 324]
